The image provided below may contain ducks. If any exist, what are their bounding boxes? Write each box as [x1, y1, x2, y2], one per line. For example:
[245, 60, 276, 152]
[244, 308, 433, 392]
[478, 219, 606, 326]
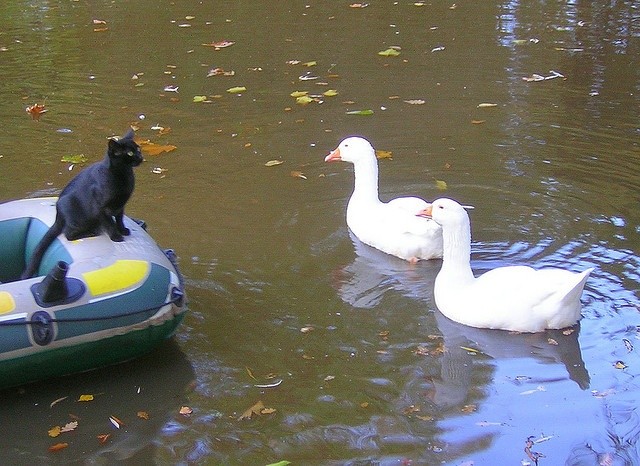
[416, 196, 596, 334]
[324, 136, 474, 265]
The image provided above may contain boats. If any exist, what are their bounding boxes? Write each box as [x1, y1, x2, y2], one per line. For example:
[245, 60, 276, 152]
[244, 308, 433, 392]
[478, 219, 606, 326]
[0, 197, 190, 391]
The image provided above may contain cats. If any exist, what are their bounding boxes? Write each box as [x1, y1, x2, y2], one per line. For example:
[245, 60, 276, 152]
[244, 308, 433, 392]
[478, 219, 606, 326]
[19, 127, 143, 282]
